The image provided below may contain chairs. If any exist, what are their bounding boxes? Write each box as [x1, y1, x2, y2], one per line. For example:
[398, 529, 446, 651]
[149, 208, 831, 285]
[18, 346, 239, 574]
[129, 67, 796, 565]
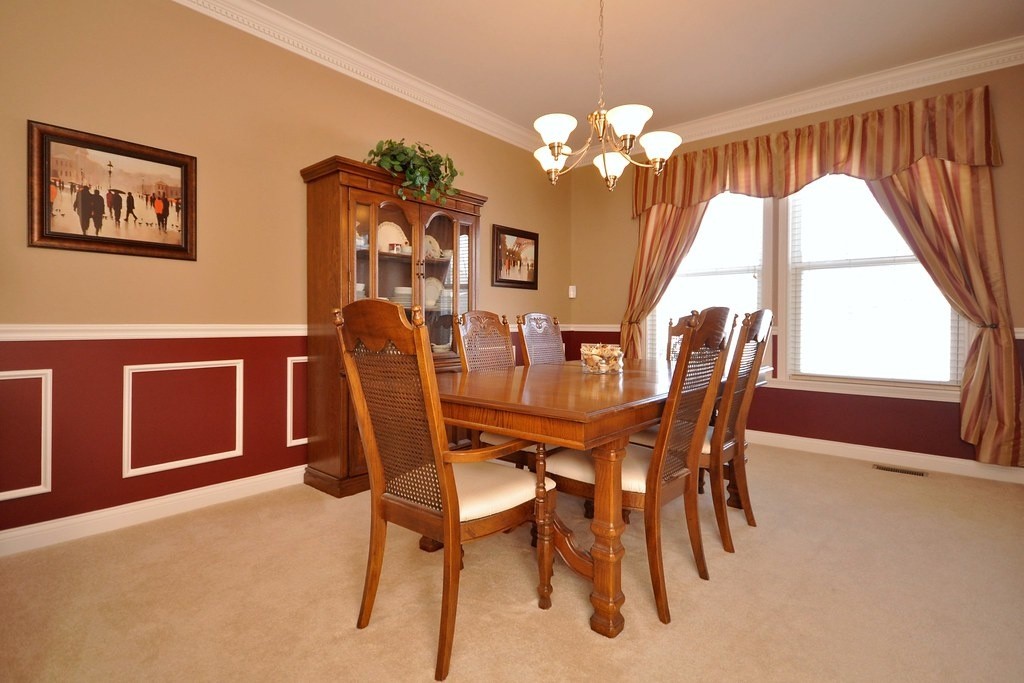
[628, 308, 774, 554]
[450, 310, 561, 548]
[546, 306, 739, 624]
[514, 311, 566, 366]
[666, 315, 692, 359]
[331, 297, 557, 681]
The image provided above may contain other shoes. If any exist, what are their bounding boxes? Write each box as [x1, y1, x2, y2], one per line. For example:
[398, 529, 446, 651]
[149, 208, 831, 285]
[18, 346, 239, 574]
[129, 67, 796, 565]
[123, 219, 128, 222]
[134, 218, 138, 221]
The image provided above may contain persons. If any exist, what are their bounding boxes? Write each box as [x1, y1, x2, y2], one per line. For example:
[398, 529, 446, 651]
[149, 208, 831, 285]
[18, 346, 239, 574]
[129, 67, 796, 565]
[73, 186, 104, 236]
[501, 257, 531, 274]
[106, 189, 126, 223]
[55, 179, 92, 195]
[124, 192, 138, 222]
[143, 190, 181, 232]
[50, 181, 58, 217]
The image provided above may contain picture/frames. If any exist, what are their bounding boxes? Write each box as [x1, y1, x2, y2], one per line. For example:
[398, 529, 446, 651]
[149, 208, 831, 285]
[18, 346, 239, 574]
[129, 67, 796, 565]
[26, 119, 197, 262]
[491, 224, 539, 290]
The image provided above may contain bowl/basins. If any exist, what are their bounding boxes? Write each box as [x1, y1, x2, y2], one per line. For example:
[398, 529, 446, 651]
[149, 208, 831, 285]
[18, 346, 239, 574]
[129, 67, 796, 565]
[394, 287, 412, 294]
[356, 284, 365, 291]
[432, 345, 451, 353]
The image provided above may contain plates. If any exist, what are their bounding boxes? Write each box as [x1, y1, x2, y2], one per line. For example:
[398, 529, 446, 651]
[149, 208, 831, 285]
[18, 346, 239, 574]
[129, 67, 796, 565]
[424, 277, 445, 306]
[378, 221, 408, 252]
[354, 290, 412, 307]
[425, 235, 440, 258]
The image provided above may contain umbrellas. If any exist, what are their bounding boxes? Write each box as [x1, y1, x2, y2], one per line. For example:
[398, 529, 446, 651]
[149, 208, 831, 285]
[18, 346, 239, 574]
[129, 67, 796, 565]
[109, 189, 126, 194]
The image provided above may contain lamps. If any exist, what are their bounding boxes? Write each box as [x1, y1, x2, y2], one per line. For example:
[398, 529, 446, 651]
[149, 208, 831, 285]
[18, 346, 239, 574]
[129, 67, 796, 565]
[532, 0, 682, 192]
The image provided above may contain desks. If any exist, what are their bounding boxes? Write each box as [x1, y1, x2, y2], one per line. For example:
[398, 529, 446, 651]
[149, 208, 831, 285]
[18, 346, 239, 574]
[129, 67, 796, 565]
[434, 358, 775, 638]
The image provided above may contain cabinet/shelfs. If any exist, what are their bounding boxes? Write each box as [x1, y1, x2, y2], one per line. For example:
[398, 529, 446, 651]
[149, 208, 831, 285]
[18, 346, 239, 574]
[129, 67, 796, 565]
[299, 153, 488, 498]
